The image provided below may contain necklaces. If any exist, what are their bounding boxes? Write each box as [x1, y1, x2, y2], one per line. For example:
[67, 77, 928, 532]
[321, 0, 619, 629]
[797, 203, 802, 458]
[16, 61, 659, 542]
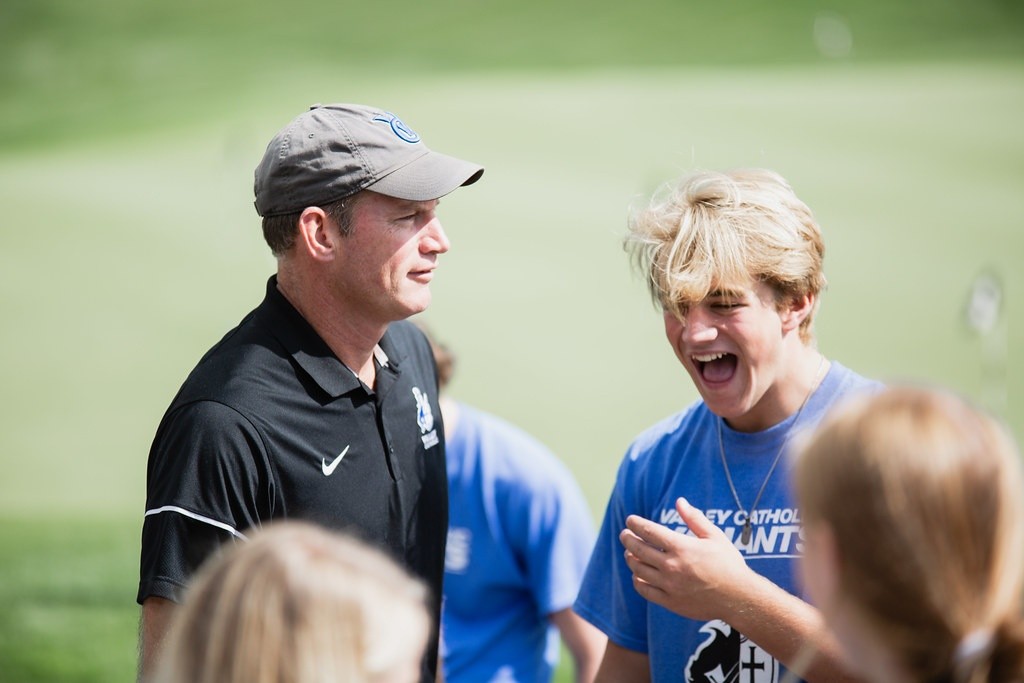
[717, 355, 825, 545]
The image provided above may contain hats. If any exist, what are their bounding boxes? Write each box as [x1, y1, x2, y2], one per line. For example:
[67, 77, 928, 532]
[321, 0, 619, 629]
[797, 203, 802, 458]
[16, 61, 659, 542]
[253, 103, 486, 219]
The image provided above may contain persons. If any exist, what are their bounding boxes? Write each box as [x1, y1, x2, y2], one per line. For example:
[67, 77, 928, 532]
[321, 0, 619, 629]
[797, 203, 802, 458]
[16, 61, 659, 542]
[406, 316, 609, 683]
[148, 519, 433, 683]
[136, 103, 486, 683]
[572, 165, 897, 683]
[791, 386, 1024, 683]
